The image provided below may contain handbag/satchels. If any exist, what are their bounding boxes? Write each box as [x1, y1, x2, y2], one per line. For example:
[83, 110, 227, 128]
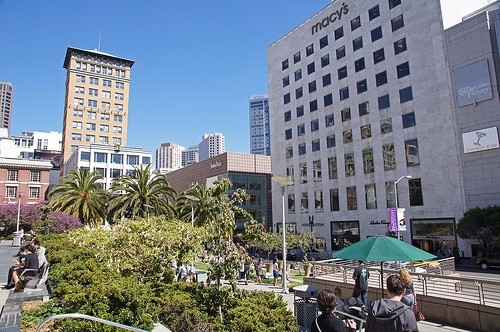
[413, 304, 425, 321]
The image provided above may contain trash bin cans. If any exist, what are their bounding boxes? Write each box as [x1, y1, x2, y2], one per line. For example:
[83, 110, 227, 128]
[293, 284, 320, 332]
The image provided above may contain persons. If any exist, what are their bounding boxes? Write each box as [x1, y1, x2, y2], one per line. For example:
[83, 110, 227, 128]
[5, 226, 39, 293]
[178, 260, 195, 283]
[310, 288, 356, 332]
[352, 261, 370, 306]
[400, 268, 417, 309]
[365, 275, 419, 332]
[241, 252, 308, 286]
[333, 288, 365, 330]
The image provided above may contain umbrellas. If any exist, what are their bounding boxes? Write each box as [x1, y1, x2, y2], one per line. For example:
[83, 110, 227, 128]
[331, 235, 438, 298]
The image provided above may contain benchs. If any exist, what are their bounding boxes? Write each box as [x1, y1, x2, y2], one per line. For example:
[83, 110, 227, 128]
[20, 261, 51, 289]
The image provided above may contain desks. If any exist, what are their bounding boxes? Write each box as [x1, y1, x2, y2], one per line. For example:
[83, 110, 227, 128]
[430, 277, 461, 292]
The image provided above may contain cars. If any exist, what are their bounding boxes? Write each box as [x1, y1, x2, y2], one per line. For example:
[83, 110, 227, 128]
[475, 253, 500, 270]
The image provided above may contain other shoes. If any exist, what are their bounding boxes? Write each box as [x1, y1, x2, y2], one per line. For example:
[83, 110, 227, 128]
[5, 284, 15, 289]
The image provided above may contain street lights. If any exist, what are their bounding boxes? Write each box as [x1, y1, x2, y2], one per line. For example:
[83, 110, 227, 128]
[142, 203, 154, 222]
[184, 194, 201, 272]
[393, 175, 412, 269]
[13, 190, 28, 236]
[270, 176, 293, 294]
[307, 215, 315, 246]
